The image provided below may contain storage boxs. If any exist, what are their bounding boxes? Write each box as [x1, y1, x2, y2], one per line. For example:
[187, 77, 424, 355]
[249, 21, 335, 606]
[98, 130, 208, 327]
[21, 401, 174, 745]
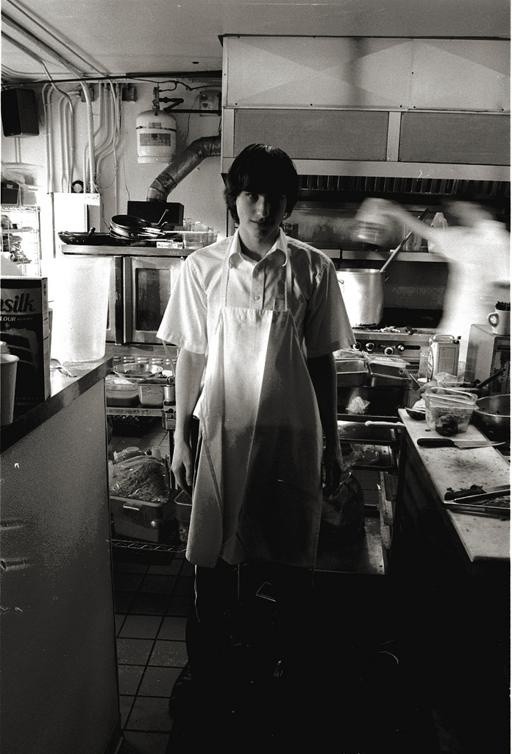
[0, 162, 44, 207]
[110, 489, 173, 544]
[172, 488, 192, 543]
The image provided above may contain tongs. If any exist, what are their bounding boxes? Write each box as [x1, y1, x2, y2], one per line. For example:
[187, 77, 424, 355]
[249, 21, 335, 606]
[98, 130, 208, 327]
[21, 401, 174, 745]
[442, 489, 509, 520]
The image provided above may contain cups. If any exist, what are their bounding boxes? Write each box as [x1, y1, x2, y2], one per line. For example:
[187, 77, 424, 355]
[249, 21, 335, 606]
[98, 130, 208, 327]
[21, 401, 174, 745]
[0, 353, 20, 427]
[486, 305, 510, 336]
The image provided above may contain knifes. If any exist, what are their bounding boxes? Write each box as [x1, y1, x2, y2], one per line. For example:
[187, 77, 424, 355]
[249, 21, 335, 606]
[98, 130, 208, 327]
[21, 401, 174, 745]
[444, 484, 509, 500]
[417, 437, 506, 449]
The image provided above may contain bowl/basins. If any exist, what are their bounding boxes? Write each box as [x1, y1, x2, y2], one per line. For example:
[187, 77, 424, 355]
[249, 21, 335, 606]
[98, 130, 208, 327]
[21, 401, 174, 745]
[353, 196, 394, 227]
[472, 393, 509, 441]
[420, 385, 481, 433]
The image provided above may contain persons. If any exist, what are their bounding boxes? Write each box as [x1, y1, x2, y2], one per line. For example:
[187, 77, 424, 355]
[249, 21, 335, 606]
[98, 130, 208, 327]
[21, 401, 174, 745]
[153, 140, 363, 718]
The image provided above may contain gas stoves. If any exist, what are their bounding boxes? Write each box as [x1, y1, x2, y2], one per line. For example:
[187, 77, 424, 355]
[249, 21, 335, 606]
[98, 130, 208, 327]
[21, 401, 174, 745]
[352, 323, 439, 334]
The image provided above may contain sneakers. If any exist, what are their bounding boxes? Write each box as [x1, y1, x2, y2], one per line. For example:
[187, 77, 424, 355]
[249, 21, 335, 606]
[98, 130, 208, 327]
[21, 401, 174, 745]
[168, 663, 216, 717]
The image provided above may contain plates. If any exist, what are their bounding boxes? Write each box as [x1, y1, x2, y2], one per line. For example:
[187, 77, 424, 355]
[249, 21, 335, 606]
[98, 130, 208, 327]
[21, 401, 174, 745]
[111, 362, 163, 378]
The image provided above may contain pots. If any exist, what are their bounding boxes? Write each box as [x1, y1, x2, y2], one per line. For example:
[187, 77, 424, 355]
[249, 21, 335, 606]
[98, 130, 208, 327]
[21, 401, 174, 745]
[107, 208, 173, 246]
[334, 267, 392, 329]
[58, 226, 110, 244]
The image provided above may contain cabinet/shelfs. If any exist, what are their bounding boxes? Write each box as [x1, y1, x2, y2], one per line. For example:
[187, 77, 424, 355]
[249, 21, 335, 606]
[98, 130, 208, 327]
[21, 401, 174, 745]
[0, 206, 42, 277]
